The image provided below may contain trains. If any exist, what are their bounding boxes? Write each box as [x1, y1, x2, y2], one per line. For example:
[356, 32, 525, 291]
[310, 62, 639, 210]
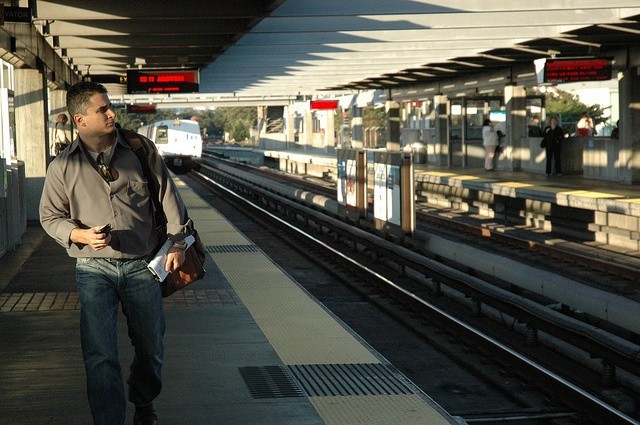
[138, 117, 202, 166]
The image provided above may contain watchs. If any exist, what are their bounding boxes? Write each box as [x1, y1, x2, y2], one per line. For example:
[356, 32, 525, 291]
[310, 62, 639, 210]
[173, 242, 188, 250]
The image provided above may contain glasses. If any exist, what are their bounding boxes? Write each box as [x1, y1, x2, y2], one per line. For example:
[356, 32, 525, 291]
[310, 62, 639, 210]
[96, 152, 108, 178]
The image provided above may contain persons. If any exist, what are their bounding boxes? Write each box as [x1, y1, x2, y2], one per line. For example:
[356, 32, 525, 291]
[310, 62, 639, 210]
[38, 82, 194, 425]
[482, 119, 499, 170]
[49, 113, 70, 158]
[528, 115, 541, 135]
[541, 116, 563, 178]
[611, 120, 620, 138]
[575, 111, 594, 136]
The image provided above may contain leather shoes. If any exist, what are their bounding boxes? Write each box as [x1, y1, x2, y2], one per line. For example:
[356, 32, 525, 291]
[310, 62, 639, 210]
[134, 413, 158, 425]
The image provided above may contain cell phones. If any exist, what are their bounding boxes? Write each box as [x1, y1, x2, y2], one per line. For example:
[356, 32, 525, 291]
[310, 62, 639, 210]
[98, 223, 111, 235]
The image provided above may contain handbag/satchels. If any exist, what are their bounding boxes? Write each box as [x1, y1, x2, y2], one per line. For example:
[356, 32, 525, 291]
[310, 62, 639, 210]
[156, 219, 210, 298]
[55, 143, 69, 156]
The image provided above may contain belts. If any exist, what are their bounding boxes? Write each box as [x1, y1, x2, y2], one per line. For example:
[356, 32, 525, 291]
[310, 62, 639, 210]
[105, 258, 128, 262]
